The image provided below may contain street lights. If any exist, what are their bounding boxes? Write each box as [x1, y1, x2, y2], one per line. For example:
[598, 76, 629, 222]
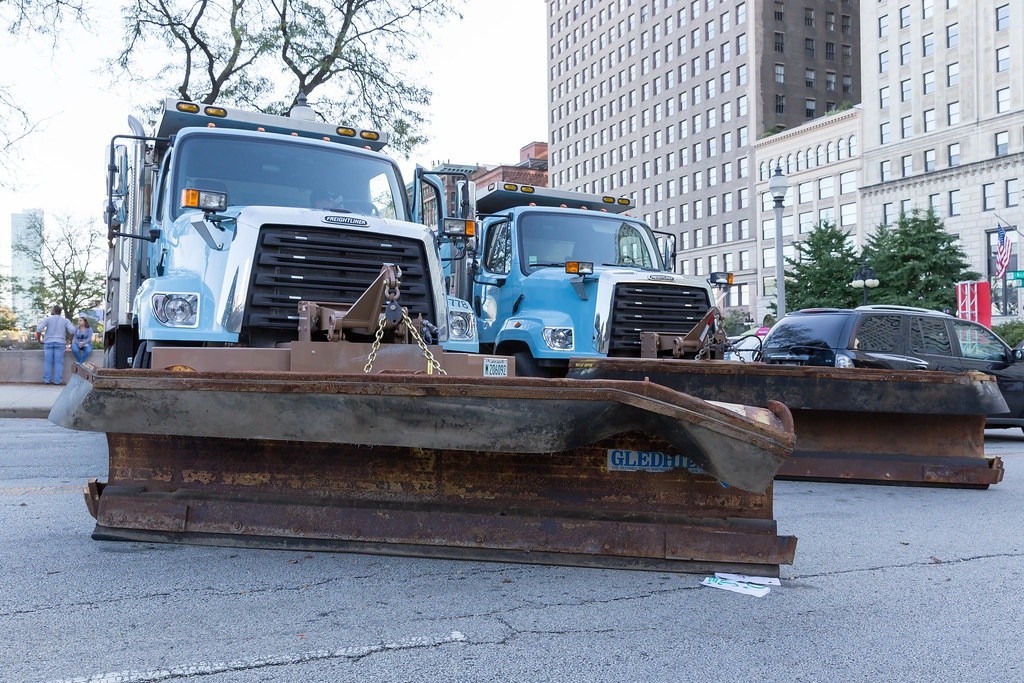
[767, 163, 790, 320]
[849, 254, 880, 305]
[743, 314, 754, 330]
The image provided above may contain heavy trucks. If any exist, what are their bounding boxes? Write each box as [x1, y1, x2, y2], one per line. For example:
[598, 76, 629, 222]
[96, 98, 486, 368]
[431, 176, 734, 378]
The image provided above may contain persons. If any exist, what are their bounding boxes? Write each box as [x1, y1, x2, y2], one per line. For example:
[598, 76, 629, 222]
[36, 305, 76, 386]
[310, 189, 340, 209]
[71, 316, 93, 364]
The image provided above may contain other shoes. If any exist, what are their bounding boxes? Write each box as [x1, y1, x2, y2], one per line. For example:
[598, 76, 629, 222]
[46, 383, 50, 384]
[54, 382, 66, 385]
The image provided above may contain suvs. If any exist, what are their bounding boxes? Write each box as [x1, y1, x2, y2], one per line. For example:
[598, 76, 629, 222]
[756, 301, 1024, 436]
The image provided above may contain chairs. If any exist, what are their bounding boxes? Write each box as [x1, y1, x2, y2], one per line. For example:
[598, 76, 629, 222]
[194, 179, 231, 205]
[572, 241, 595, 260]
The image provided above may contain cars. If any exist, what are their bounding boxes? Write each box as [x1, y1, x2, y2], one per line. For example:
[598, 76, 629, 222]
[722, 335, 766, 361]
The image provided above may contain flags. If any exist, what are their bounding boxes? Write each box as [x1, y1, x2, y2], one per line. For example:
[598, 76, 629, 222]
[995, 220, 1013, 281]
